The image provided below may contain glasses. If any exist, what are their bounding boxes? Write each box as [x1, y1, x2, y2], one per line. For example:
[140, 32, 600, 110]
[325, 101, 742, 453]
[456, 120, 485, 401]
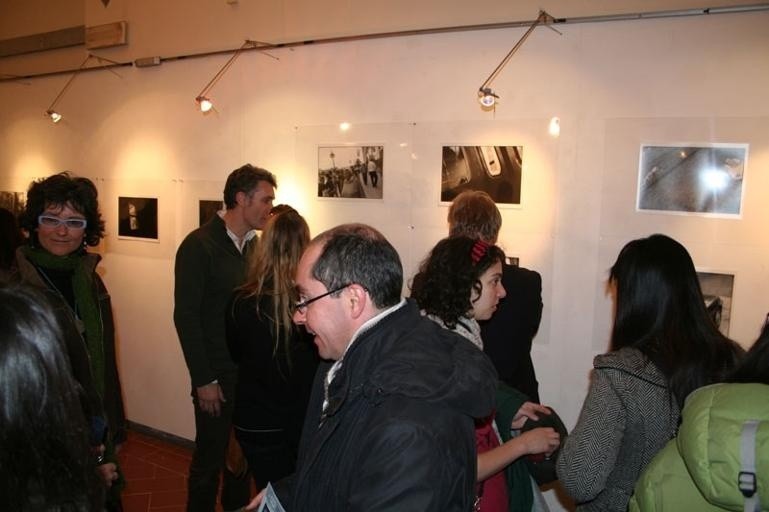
[291, 283, 367, 314]
[39, 216, 87, 230]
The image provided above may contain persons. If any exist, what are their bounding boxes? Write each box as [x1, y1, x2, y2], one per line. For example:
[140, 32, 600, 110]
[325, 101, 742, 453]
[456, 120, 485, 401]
[14, 173, 127, 457]
[243, 223, 498, 511]
[411, 236, 560, 512]
[0, 287, 119, 512]
[554, 236, 747, 512]
[628, 311, 769, 512]
[318, 158, 380, 196]
[173, 163, 278, 512]
[449, 191, 543, 406]
[225, 204, 320, 495]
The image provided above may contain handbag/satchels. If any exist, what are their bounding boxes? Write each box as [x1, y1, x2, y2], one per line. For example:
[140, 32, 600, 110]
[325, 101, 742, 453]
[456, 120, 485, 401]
[520, 406, 570, 486]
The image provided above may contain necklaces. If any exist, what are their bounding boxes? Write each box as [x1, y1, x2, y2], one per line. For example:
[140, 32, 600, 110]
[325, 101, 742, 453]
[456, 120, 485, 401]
[38, 266, 79, 319]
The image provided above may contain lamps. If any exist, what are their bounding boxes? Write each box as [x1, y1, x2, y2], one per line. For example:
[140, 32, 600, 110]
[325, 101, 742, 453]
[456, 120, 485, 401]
[479, 10, 557, 112]
[44, 53, 122, 123]
[194, 40, 280, 113]
